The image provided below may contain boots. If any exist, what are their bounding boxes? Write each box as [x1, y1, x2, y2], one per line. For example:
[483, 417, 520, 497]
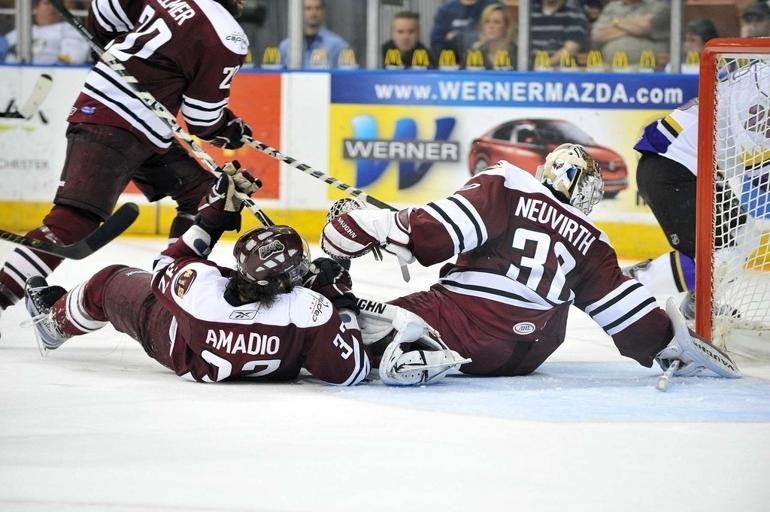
[24, 276, 70, 350]
[621, 258, 655, 282]
[679, 292, 741, 321]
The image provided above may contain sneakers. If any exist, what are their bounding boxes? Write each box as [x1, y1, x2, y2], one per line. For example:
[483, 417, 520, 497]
[390, 349, 463, 386]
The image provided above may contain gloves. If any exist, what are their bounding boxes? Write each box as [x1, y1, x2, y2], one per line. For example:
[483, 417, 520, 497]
[197, 160, 263, 231]
[199, 108, 253, 149]
[317, 206, 416, 283]
[302, 258, 361, 313]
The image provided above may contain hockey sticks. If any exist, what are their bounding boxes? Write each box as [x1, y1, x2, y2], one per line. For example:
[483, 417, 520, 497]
[0, 75, 51, 119]
[1, 203, 139, 260]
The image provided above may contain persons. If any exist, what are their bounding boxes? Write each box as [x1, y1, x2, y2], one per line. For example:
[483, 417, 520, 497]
[24, 161, 373, 387]
[0, 1, 253, 341]
[622, 58, 770, 314]
[728, 0, 768, 73]
[382, 1, 517, 69]
[274, 1, 354, 65]
[2, 0, 92, 66]
[529, 1, 718, 66]
[321, 143, 743, 387]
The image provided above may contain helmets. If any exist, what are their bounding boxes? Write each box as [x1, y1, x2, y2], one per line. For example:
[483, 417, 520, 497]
[536, 142, 605, 214]
[233, 225, 313, 287]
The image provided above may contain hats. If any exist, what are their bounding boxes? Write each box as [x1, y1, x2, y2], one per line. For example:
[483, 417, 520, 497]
[741, 0, 770, 19]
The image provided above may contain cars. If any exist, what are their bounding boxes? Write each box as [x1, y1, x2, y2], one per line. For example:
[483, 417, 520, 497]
[466, 115, 630, 204]
